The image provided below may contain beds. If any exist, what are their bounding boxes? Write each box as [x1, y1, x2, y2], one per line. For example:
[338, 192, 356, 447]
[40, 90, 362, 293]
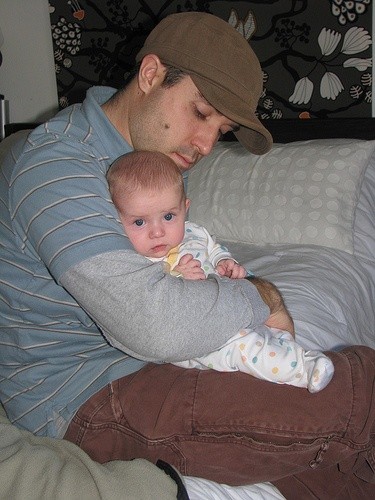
[0, 123, 375, 500]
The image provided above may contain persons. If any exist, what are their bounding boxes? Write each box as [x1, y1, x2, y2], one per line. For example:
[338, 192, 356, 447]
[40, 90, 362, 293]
[0, 11, 375, 500]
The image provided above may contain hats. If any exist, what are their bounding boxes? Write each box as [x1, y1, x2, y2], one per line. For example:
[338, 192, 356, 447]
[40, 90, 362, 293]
[136, 10, 273, 157]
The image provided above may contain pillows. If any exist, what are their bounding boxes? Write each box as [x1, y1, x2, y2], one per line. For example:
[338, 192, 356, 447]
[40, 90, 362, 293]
[185, 138, 375, 255]
